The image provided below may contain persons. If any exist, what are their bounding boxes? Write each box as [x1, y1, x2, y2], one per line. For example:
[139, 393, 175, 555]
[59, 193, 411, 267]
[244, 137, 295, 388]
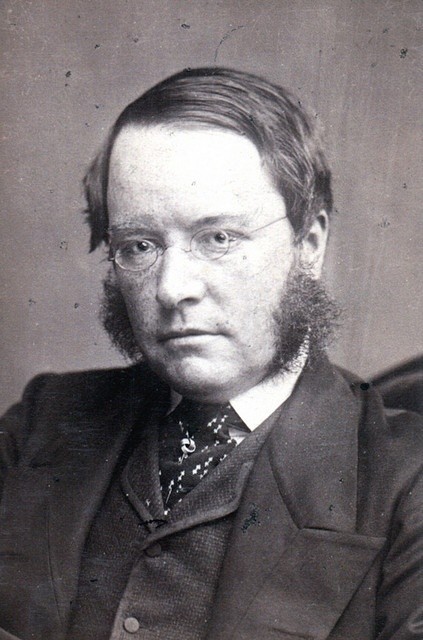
[0, 67, 421, 640]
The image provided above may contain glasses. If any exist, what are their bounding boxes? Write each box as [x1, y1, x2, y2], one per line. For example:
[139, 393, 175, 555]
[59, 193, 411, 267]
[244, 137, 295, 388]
[104, 211, 308, 274]
